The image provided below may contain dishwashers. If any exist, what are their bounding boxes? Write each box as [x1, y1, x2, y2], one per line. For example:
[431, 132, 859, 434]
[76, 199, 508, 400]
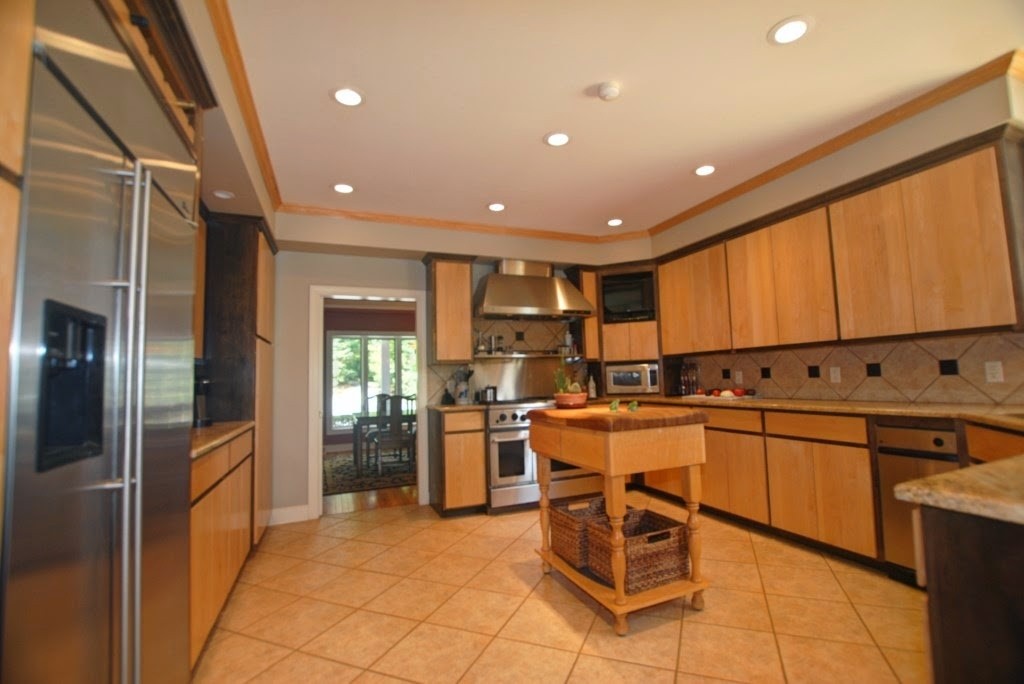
[876, 424, 962, 572]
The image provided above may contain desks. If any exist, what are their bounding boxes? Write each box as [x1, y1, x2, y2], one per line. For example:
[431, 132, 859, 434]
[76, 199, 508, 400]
[351, 411, 416, 479]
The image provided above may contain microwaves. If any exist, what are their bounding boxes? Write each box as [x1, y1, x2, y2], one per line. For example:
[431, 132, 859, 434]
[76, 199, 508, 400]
[606, 362, 659, 394]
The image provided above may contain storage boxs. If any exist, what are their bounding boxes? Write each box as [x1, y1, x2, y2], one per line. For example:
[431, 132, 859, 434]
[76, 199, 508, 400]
[548, 491, 634, 570]
[586, 507, 686, 594]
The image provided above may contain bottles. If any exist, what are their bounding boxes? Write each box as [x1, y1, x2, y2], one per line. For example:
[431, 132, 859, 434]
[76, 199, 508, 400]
[490, 335, 495, 354]
[588, 375, 597, 398]
[194, 357, 212, 426]
[498, 335, 503, 351]
[565, 331, 578, 355]
[477, 330, 486, 352]
[680, 362, 698, 395]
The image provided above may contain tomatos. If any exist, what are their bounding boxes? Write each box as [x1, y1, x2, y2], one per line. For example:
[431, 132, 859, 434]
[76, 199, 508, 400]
[696, 388, 705, 394]
[712, 389, 720, 397]
[733, 389, 744, 396]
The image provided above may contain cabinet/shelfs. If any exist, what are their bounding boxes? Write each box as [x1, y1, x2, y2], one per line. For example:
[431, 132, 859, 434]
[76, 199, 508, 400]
[826, 141, 1024, 344]
[566, 270, 601, 360]
[207, 220, 274, 544]
[765, 412, 878, 564]
[601, 322, 658, 363]
[642, 406, 768, 527]
[428, 411, 488, 516]
[724, 203, 839, 352]
[426, 256, 473, 365]
[527, 403, 710, 637]
[191, 428, 252, 672]
[656, 240, 732, 359]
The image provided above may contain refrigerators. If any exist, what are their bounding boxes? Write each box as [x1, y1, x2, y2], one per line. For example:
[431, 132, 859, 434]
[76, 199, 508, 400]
[0, 0, 200, 684]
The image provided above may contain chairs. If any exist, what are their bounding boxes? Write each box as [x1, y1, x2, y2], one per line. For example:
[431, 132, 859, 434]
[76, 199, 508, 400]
[365, 394, 415, 475]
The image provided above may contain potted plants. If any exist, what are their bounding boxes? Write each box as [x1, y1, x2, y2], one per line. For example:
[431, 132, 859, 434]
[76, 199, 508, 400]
[553, 358, 587, 409]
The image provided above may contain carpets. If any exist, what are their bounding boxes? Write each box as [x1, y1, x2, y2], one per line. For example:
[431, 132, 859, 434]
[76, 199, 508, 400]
[322, 448, 416, 495]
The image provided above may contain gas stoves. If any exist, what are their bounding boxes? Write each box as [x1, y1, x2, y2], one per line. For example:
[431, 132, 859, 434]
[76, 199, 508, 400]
[479, 400, 557, 432]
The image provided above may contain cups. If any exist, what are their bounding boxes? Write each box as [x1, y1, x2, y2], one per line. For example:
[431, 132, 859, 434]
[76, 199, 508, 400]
[482, 386, 496, 402]
[454, 381, 469, 404]
[557, 345, 570, 354]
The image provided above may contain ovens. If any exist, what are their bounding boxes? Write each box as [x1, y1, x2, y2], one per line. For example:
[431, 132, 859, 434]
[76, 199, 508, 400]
[486, 431, 604, 508]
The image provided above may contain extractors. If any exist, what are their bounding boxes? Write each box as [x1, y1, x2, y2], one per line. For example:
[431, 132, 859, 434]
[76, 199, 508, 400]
[472, 258, 596, 322]
